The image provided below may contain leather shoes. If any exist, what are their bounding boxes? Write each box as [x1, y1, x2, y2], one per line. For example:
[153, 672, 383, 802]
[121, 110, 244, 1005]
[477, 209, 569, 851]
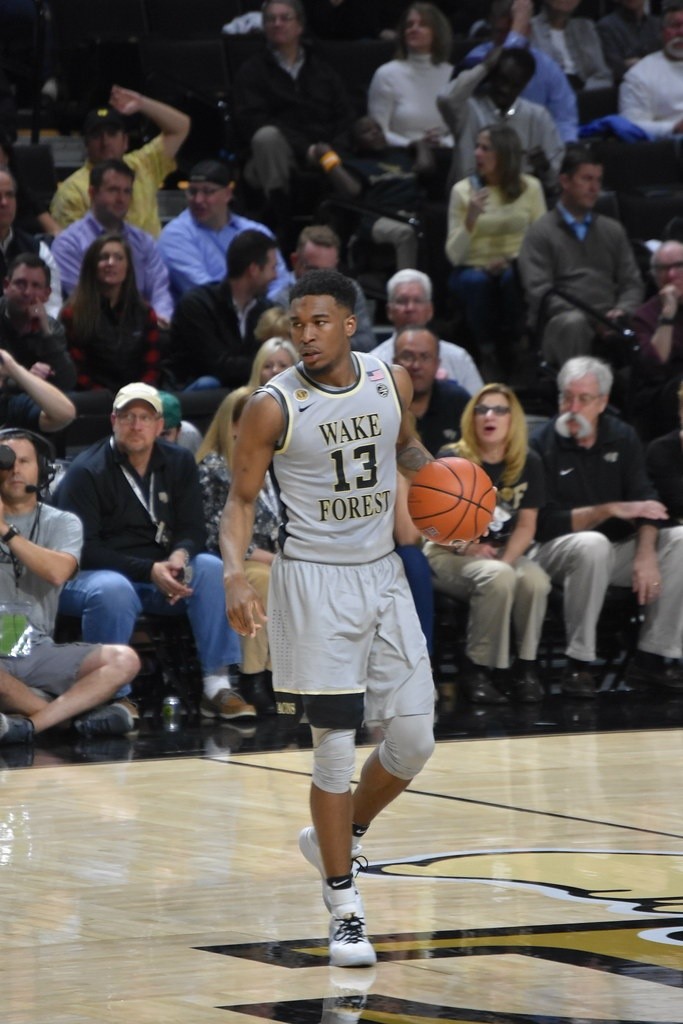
[458, 677, 510, 705]
[238, 680, 277, 714]
[511, 664, 540, 703]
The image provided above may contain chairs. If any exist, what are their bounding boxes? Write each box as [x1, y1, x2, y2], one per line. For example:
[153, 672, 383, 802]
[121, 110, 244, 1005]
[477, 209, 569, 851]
[126, 591, 654, 726]
[0, 0, 402, 229]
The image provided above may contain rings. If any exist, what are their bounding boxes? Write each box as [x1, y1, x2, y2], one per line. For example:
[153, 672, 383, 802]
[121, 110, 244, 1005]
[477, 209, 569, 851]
[168, 594, 173, 598]
[652, 582, 659, 586]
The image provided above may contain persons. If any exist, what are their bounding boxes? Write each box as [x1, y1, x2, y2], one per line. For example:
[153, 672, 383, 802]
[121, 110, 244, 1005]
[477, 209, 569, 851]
[197, 385, 283, 714]
[51, 381, 257, 720]
[527, 356, 683, 699]
[0, 1, 683, 689]
[421, 384, 552, 704]
[219, 268, 499, 967]
[0, 427, 143, 742]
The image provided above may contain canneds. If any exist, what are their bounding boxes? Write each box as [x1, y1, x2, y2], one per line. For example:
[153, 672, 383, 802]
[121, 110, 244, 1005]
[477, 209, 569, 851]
[163, 696, 181, 730]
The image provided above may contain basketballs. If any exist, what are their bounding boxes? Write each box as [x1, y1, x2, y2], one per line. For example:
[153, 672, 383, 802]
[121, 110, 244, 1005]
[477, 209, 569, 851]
[407, 456, 496, 547]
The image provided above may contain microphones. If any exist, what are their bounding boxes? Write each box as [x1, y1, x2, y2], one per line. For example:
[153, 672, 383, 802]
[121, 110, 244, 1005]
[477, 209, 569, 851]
[25, 474, 55, 494]
[0, 444, 17, 470]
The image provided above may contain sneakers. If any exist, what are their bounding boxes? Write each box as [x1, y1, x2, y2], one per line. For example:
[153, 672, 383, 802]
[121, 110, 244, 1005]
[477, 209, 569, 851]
[200, 720, 257, 751]
[110, 697, 140, 718]
[327, 914, 377, 967]
[298, 825, 361, 915]
[72, 733, 133, 763]
[322, 968, 376, 1017]
[199, 690, 257, 720]
[74, 703, 135, 735]
[0, 712, 35, 745]
[0, 745, 34, 769]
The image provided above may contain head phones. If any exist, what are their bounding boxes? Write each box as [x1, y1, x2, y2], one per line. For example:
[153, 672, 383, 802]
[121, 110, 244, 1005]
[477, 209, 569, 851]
[0, 427, 57, 489]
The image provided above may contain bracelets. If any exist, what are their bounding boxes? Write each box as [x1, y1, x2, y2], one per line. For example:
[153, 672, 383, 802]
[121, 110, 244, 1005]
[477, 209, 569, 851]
[454, 540, 473, 555]
[177, 548, 189, 566]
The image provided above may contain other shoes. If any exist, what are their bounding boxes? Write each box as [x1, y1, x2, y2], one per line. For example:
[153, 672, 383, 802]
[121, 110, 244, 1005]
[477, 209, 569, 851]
[561, 672, 592, 698]
[624, 662, 683, 693]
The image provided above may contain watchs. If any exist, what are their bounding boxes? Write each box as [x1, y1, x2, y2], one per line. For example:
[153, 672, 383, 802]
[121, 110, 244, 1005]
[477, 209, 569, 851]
[1, 524, 20, 542]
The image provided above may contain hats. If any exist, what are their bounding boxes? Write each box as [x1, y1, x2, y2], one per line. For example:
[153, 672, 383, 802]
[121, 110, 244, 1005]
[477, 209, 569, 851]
[188, 161, 231, 186]
[112, 381, 163, 420]
[155, 390, 182, 429]
[84, 107, 128, 132]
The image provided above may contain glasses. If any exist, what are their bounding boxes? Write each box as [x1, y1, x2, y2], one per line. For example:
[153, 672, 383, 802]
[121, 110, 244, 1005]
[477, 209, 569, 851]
[655, 262, 683, 270]
[471, 404, 510, 415]
[399, 352, 434, 367]
[559, 392, 601, 403]
[117, 411, 155, 425]
